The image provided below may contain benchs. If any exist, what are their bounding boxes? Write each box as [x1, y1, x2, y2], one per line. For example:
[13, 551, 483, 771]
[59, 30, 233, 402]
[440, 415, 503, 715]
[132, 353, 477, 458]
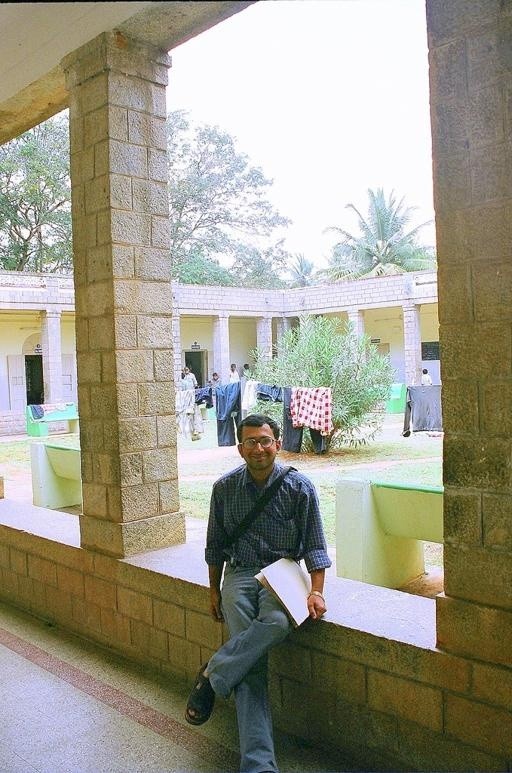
[26, 402, 82, 509]
[334, 478, 443, 591]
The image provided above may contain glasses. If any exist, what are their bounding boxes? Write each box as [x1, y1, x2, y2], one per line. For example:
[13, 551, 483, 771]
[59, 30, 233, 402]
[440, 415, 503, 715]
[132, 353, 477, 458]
[237, 436, 277, 449]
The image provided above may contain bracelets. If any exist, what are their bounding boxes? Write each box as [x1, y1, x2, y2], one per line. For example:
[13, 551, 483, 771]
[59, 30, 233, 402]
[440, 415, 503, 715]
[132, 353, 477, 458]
[307, 590, 326, 602]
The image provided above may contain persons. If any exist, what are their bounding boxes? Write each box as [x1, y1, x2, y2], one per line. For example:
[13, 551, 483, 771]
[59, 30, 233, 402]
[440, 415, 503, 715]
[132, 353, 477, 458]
[186, 415, 333, 773]
[420, 367, 432, 385]
[227, 363, 240, 383]
[242, 362, 255, 381]
[182, 366, 197, 389]
[206, 372, 223, 387]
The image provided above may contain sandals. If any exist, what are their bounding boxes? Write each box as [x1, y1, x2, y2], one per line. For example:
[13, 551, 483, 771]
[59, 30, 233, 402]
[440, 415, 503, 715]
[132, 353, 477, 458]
[183, 661, 218, 725]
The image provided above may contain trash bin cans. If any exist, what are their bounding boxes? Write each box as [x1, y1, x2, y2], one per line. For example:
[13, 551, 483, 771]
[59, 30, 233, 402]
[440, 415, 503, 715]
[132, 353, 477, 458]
[386, 383, 407, 414]
